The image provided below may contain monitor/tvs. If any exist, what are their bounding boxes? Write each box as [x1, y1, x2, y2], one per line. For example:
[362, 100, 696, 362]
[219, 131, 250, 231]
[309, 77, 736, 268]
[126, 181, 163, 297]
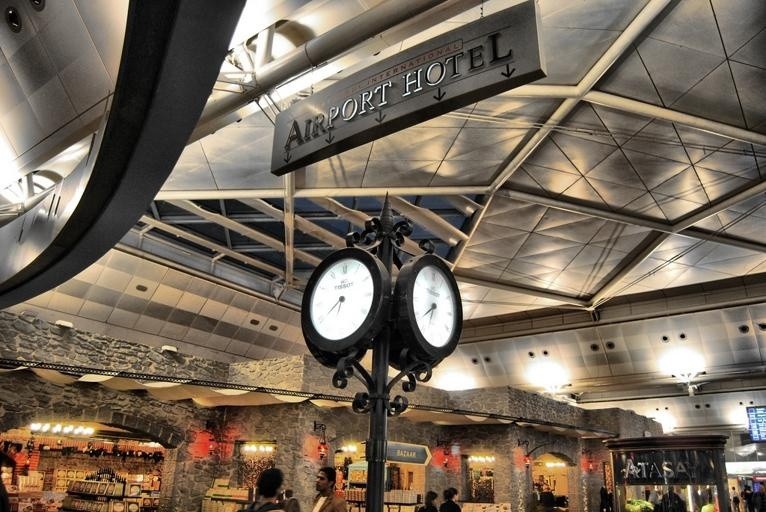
[746, 405, 766, 443]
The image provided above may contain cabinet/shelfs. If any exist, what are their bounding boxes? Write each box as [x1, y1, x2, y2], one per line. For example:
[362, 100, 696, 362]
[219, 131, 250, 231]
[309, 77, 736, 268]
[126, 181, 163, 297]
[345, 488, 422, 512]
[202, 486, 253, 512]
[57, 479, 161, 512]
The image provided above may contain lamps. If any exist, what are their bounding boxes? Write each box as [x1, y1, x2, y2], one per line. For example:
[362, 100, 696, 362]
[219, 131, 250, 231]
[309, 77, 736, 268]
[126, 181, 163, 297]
[38, 442, 163, 462]
[589, 464, 593, 472]
[313, 420, 327, 446]
[24, 460, 30, 470]
[443, 458, 448, 467]
[525, 459, 530, 468]
[320, 448, 325, 459]
[437, 439, 449, 455]
[582, 448, 593, 463]
[24, 437, 35, 456]
[518, 439, 529, 456]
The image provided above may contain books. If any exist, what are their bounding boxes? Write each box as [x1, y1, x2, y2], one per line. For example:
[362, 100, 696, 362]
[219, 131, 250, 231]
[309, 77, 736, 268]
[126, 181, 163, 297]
[56, 468, 162, 512]
[336, 485, 420, 503]
[1, 466, 56, 511]
[201, 484, 251, 511]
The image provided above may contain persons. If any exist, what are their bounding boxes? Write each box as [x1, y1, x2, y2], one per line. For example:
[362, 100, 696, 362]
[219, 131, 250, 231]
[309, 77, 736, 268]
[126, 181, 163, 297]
[418, 491, 438, 511]
[311, 467, 347, 512]
[662, 486, 685, 511]
[245, 468, 286, 512]
[283, 489, 299, 511]
[440, 487, 462, 512]
[732, 480, 766, 512]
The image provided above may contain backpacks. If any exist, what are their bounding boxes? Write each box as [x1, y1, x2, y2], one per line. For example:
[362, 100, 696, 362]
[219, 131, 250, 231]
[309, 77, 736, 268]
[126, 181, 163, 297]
[236, 500, 281, 512]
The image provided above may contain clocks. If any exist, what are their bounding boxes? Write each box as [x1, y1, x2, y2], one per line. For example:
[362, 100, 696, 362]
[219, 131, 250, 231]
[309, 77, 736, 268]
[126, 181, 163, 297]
[300, 247, 391, 352]
[395, 254, 463, 362]
